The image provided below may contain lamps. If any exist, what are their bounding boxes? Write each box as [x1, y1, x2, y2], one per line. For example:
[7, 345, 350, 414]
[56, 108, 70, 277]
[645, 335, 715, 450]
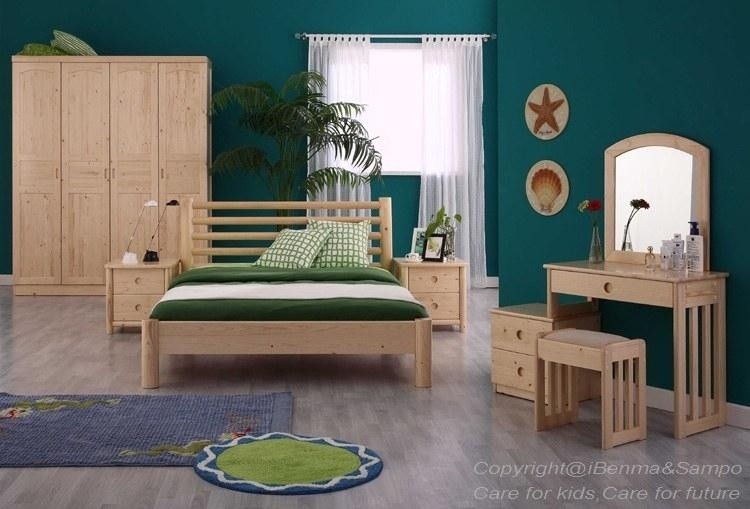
[121, 199, 159, 266]
[142, 199, 181, 264]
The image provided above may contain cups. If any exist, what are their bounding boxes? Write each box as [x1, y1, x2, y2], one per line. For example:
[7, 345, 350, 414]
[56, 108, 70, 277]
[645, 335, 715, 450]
[404, 252, 420, 260]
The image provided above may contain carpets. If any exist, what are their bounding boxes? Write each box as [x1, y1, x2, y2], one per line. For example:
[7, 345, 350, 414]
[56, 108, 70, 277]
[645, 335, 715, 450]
[0, 393, 293, 468]
[192, 430, 382, 496]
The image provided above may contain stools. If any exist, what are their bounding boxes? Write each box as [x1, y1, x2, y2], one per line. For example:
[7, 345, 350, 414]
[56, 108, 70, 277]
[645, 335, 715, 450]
[533, 328, 647, 450]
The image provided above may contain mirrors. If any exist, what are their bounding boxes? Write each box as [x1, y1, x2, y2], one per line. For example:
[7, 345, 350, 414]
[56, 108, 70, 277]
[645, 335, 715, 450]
[604, 131, 710, 271]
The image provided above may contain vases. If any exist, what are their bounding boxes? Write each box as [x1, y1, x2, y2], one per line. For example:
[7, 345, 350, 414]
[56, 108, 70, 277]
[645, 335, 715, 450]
[587, 225, 604, 265]
[440, 232, 455, 260]
[623, 224, 633, 251]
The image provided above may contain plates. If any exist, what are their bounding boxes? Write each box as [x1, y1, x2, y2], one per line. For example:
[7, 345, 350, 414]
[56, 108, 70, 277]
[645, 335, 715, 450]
[406, 258, 424, 263]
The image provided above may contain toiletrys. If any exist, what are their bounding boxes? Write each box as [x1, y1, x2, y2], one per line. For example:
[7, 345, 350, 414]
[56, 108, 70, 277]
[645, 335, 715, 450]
[667, 239, 674, 270]
[645, 246, 656, 272]
[678, 254, 685, 270]
[672, 234, 684, 270]
[659, 240, 669, 271]
[685, 221, 704, 272]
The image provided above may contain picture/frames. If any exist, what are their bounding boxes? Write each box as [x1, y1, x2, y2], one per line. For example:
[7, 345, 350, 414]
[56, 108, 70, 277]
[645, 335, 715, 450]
[421, 232, 446, 264]
[409, 227, 430, 260]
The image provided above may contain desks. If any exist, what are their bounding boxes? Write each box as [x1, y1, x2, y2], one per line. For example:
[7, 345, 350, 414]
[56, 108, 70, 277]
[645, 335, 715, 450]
[488, 261, 729, 440]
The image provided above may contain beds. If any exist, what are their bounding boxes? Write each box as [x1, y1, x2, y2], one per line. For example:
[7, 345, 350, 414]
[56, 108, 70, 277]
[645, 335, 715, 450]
[140, 197, 432, 388]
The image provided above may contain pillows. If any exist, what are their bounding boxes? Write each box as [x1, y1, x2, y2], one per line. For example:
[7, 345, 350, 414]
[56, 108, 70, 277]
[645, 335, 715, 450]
[251, 224, 331, 270]
[304, 216, 375, 271]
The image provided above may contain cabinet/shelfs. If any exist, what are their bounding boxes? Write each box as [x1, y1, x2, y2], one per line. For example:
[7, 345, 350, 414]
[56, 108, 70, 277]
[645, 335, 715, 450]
[158, 55, 213, 277]
[110, 55, 160, 265]
[12, 55, 61, 297]
[61, 56, 110, 296]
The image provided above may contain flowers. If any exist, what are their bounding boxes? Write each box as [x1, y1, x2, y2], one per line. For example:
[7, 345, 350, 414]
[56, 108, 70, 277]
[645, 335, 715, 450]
[621, 198, 650, 250]
[425, 207, 461, 237]
[578, 197, 604, 258]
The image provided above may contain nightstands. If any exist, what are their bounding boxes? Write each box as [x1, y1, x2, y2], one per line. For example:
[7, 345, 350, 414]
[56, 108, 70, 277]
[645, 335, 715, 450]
[393, 256, 468, 334]
[105, 261, 179, 336]
